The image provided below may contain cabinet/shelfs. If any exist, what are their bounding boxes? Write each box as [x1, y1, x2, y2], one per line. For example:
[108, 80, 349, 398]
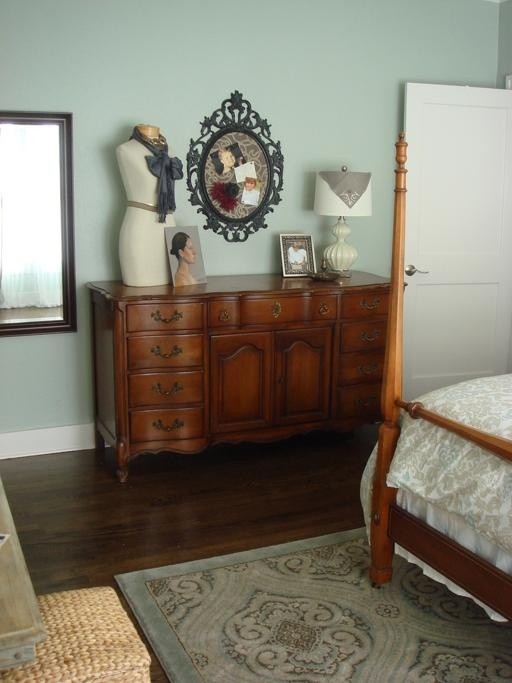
[89, 269, 407, 485]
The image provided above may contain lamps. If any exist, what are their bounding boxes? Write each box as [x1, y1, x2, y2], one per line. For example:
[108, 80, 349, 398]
[312, 163, 374, 280]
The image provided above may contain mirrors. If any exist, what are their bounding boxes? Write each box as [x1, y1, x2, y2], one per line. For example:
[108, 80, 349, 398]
[0, 109, 78, 337]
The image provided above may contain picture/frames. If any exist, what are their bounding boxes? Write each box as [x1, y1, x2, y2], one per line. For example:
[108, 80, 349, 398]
[184, 89, 284, 246]
[279, 231, 319, 279]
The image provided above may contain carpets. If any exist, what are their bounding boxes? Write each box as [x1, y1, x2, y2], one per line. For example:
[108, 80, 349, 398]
[115, 523, 511, 682]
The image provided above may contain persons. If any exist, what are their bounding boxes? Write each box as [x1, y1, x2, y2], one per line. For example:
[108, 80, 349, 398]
[242, 177, 259, 205]
[170, 232, 198, 287]
[116, 124, 176, 287]
[218, 149, 237, 173]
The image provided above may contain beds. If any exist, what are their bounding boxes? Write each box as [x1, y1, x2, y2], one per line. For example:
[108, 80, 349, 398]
[365, 131, 512, 622]
